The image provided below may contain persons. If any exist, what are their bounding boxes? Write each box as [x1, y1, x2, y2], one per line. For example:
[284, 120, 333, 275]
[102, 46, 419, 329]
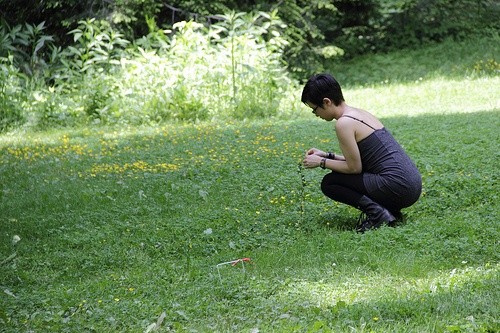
[301, 72, 422, 234]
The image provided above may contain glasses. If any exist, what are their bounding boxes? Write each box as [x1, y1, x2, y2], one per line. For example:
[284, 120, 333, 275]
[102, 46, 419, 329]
[312, 99, 323, 114]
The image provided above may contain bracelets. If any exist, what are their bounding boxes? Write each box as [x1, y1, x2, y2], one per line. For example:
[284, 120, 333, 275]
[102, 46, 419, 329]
[326, 152, 334, 159]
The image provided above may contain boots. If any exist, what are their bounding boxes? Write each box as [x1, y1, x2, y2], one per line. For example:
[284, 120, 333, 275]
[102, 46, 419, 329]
[356, 195, 396, 234]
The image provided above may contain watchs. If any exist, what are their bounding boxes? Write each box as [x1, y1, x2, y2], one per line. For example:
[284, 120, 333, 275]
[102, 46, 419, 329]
[320, 158, 326, 169]
